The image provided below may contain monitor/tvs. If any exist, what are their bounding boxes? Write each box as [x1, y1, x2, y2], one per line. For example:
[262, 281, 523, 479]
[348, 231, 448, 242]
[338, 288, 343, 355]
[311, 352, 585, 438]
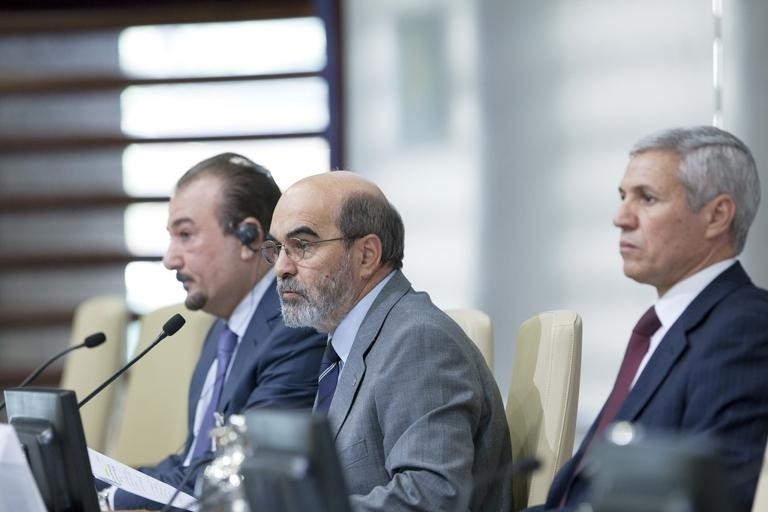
[4, 388, 101, 512]
[243, 410, 354, 512]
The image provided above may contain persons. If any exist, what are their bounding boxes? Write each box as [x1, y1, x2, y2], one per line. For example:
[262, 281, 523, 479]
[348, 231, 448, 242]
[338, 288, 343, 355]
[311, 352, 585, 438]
[94, 153, 328, 512]
[266, 169, 511, 512]
[516, 123, 767, 512]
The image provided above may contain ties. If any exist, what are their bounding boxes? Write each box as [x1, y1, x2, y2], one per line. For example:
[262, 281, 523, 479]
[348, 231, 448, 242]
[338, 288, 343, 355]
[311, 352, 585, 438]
[558, 305, 662, 511]
[193, 324, 238, 468]
[314, 337, 343, 423]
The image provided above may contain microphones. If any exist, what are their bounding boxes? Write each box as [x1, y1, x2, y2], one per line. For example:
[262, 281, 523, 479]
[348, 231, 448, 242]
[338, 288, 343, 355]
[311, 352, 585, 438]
[0, 332, 106, 411]
[76, 312, 186, 408]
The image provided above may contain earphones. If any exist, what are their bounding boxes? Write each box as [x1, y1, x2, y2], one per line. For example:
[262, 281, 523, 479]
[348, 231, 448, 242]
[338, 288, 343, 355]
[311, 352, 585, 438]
[236, 225, 258, 244]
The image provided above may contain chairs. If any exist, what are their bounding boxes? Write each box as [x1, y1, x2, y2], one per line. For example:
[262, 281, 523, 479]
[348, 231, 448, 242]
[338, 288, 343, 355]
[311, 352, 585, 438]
[504, 307, 581, 511]
[113, 307, 216, 472]
[58, 296, 125, 454]
[438, 308, 490, 371]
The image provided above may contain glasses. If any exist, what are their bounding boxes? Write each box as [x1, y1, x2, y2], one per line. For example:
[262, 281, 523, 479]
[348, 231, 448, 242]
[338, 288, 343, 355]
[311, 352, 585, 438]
[260, 237, 358, 264]
